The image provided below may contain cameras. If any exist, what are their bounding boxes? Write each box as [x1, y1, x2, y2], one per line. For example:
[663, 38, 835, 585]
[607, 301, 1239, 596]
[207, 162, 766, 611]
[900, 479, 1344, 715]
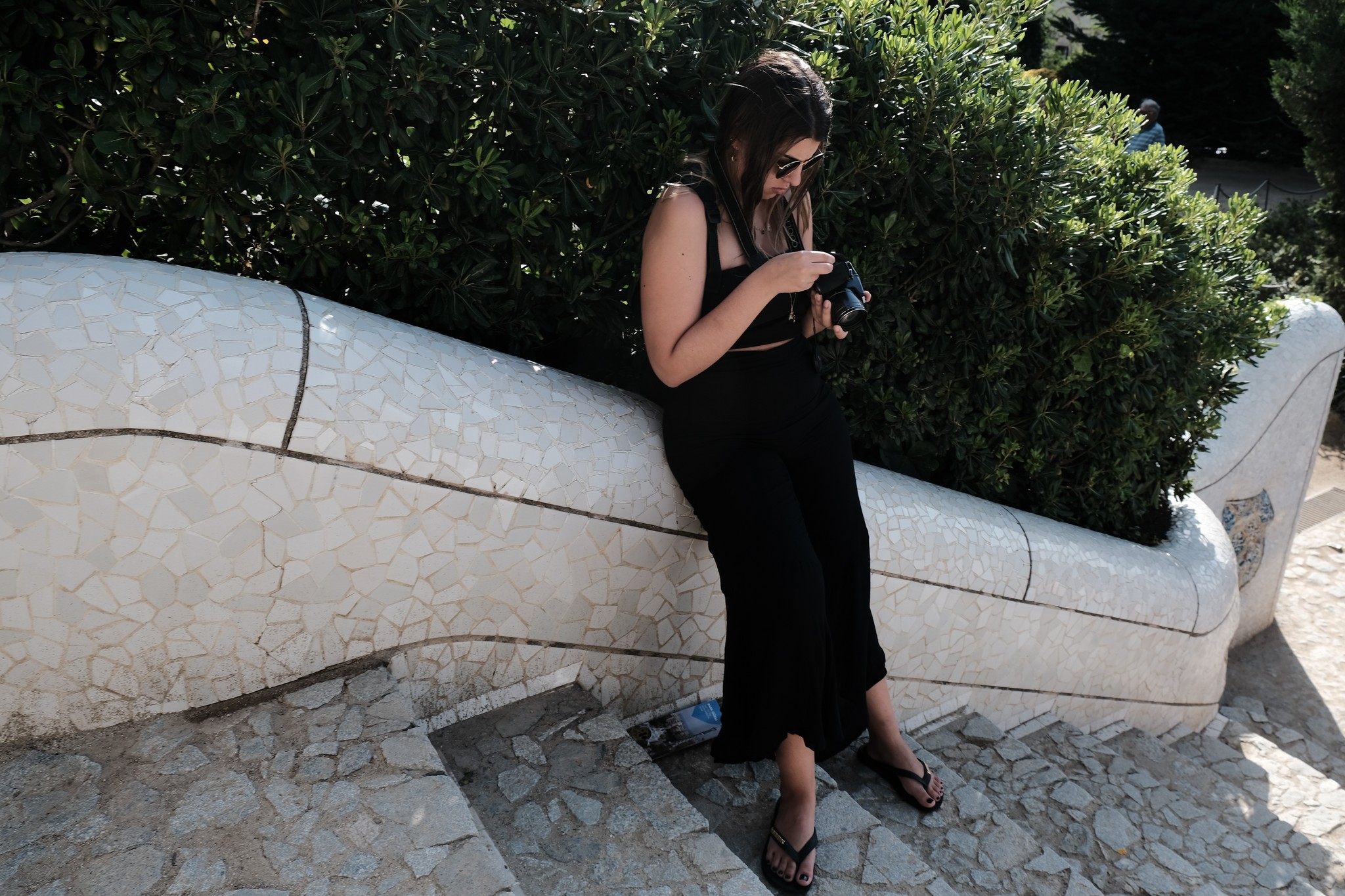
[810, 249, 868, 334]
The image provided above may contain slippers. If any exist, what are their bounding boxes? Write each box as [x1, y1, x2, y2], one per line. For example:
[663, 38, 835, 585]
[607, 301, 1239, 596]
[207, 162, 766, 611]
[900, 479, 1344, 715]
[761, 794, 818, 896]
[857, 741, 944, 814]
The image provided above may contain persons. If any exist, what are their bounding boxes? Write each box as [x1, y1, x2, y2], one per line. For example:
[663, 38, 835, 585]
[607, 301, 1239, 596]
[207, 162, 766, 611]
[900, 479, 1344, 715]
[639, 52, 946, 896]
[1123, 100, 1165, 155]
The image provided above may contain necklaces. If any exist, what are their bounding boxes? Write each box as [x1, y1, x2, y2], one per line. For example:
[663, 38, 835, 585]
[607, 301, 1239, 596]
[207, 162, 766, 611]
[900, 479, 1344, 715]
[751, 218, 770, 234]
[753, 225, 798, 323]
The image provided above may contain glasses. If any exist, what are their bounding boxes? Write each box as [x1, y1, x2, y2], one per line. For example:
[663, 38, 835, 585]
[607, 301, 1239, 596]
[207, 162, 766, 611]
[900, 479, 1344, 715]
[774, 146, 826, 180]
